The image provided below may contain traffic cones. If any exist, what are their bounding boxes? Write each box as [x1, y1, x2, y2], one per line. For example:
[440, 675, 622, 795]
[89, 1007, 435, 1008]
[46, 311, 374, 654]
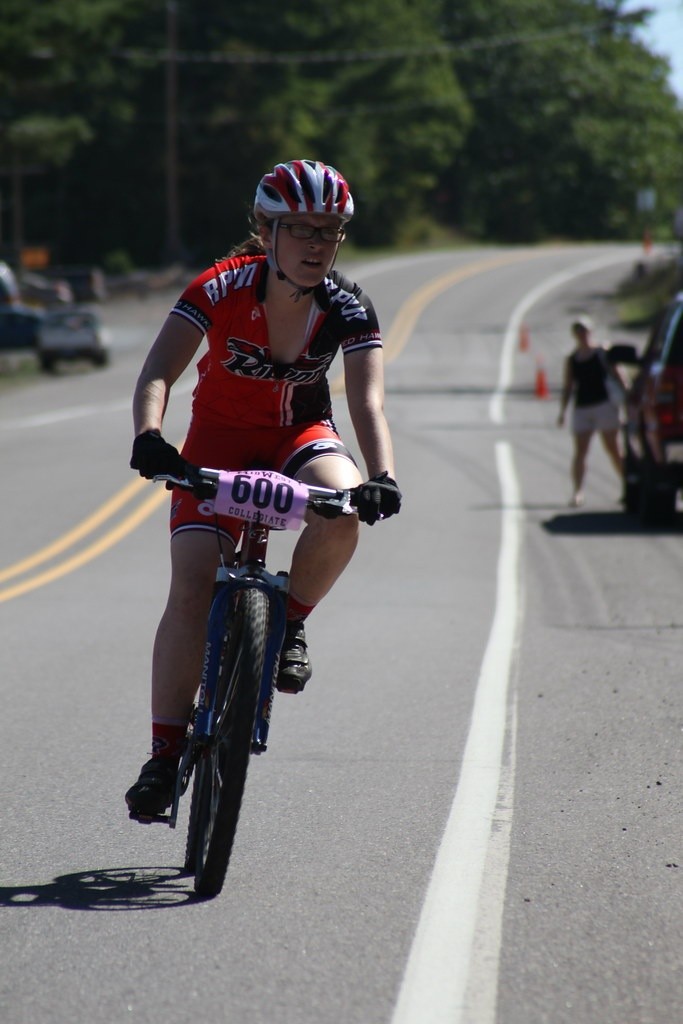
[534, 358, 550, 397]
[519, 320, 532, 353]
[643, 227, 654, 256]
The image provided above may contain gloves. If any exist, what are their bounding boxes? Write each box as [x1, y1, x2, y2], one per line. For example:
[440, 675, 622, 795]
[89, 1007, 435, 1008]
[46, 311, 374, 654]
[355, 471, 402, 526]
[130, 429, 182, 490]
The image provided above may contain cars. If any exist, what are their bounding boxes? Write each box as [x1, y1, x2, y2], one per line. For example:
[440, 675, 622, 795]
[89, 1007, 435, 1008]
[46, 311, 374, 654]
[607, 292, 683, 527]
[36, 305, 111, 373]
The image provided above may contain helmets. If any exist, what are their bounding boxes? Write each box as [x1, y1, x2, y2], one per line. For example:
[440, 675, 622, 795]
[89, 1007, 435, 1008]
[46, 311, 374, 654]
[254, 159, 354, 225]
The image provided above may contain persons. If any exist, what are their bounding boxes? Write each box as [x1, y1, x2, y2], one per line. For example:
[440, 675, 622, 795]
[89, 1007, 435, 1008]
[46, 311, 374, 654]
[125, 160, 403, 810]
[558, 324, 629, 507]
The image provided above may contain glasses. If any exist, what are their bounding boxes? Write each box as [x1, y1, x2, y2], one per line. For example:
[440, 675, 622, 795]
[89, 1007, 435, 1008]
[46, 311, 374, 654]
[264, 221, 344, 242]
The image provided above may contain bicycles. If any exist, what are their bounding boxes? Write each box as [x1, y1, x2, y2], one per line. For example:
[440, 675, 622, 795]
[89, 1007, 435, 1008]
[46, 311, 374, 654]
[130, 461, 385, 898]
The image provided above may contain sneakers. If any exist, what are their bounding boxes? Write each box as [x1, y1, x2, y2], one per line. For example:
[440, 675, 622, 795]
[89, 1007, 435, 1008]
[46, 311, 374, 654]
[125, 756, 177, 813]
[276, 620, 311, 691]
